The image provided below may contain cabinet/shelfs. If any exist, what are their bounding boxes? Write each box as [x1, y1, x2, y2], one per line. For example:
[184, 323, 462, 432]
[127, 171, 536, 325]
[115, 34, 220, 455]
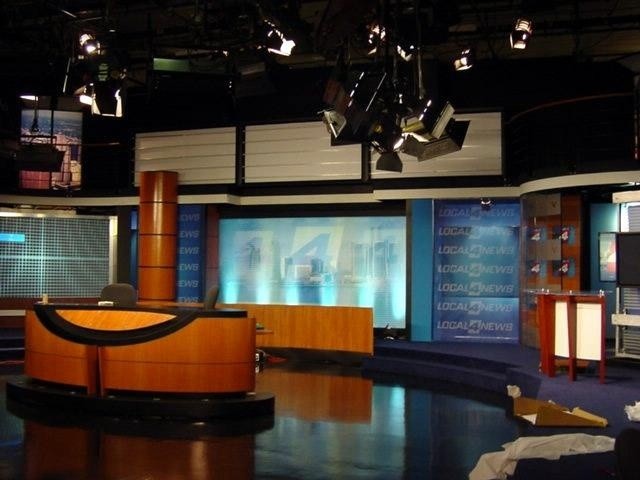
[520, 189, 581, 350]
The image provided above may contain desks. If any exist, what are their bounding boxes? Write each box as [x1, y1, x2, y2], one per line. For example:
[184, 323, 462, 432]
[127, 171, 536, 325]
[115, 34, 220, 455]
[25, 301, 257, 400]
[529, 287, 612, 385]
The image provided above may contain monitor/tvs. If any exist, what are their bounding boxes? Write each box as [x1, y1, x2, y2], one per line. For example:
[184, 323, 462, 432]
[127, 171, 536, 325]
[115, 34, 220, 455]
[8, 92, 88, 193]
[614, 231, 640, 288]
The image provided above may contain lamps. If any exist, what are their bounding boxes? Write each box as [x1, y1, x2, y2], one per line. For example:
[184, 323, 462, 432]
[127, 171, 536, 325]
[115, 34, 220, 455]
[320, 23, 476, 174]
[510, 17, 534, 51]
[265, 28, 297, 57]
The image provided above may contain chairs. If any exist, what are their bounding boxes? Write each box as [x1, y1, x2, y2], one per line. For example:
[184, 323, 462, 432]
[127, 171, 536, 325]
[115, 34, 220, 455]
[101, 283, 137, 305]
[204, 285, 220, 308]
